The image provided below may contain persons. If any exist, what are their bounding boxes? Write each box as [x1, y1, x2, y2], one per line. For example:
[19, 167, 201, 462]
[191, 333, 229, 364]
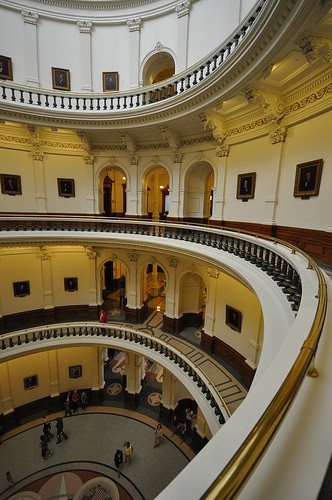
[113, 449, 123, 475]
[39, 435, 53, 461]
[55, 417, 68, 444]
[42, 417, 54, 442]
[153, 424, 162, 448]
[123, 441, 133, 465]
[119, 285, 125, 307]
[99, 306, 107, 323]
[64, 389, 87, 416]
[6, 471, 15, 484]
[185, 407, 193, 430]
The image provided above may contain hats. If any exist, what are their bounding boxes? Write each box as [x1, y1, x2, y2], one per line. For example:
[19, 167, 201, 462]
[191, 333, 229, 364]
[56, 417, 62, 420]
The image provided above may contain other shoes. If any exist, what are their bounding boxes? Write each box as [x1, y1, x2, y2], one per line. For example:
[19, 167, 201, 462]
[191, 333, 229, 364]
[153, 444, 156, 448]
[157, 444, 160, 448]
[40, 457, 47, 461]
[47, 450, 55, 456]
[65, 436, 68, 440]
[116, 461, 130, 478]
[64, 408, 88, 417]
[56, 440, 62, 444]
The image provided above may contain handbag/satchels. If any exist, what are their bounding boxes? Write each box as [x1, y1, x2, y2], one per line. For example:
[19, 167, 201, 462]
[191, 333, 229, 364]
[40, 435, 51, 443]
